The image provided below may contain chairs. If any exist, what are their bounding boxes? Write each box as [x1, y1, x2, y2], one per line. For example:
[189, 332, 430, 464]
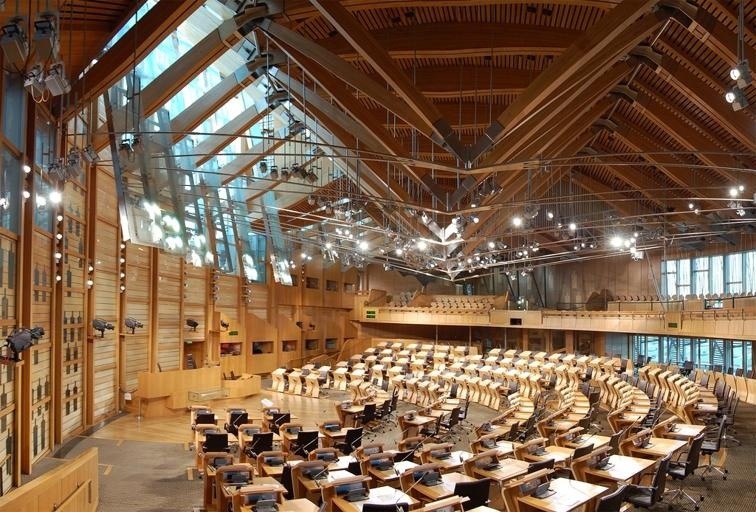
[182, 286, 756, 512]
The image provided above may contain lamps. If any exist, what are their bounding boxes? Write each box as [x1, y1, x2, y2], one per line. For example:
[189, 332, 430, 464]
[303, 123, 749, 283]
[726, 1, 751, 113]
[242, 21, 323, 184]
[1, 1, 143, 186]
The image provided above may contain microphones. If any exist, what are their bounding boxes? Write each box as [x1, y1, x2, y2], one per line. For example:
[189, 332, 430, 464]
[351, 429, 369, 452]
[274, 411, 291, 432]
[248, 435, 262, 458]
[394, 470, 432, 512]
[578, 431, 598, 450]
[619, 416, 642, 441]
[652, 403, 676, 433]
[302, 435, 321, 457]
[400, 442, 422, 464]
[233, 411, 247, 429]
[418, 432, 440, 450]
[313, 458, 342, 487]
